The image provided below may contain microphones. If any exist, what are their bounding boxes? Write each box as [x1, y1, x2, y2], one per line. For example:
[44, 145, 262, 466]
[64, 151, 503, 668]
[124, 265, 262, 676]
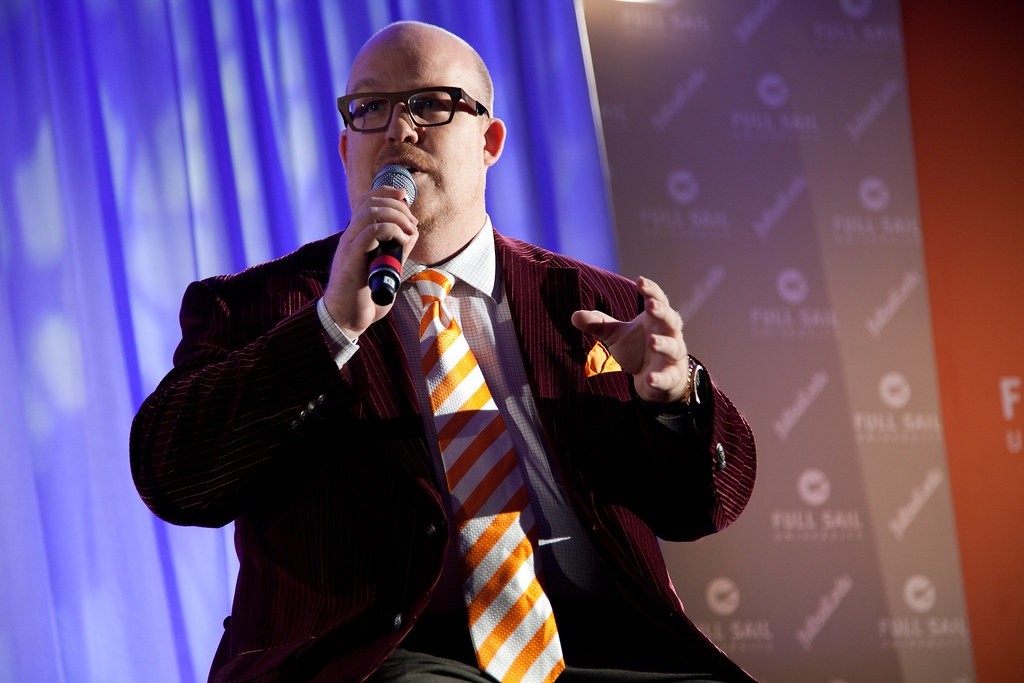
[369, 164, 417, 306]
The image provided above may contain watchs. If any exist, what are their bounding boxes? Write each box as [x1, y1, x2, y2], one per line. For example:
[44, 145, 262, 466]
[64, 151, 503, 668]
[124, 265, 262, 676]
[677, 358, 705, 412]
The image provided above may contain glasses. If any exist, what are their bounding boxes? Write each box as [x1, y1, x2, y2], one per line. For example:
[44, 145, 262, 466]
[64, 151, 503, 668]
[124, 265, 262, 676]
[336, 86, 489, 133]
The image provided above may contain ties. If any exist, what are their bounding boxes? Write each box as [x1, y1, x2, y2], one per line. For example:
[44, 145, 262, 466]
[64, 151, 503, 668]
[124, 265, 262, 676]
[404, 266, 567, 683]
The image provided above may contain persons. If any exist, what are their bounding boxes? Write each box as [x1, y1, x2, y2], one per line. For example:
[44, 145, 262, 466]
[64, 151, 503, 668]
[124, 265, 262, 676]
[129, 21, 759, 683]
